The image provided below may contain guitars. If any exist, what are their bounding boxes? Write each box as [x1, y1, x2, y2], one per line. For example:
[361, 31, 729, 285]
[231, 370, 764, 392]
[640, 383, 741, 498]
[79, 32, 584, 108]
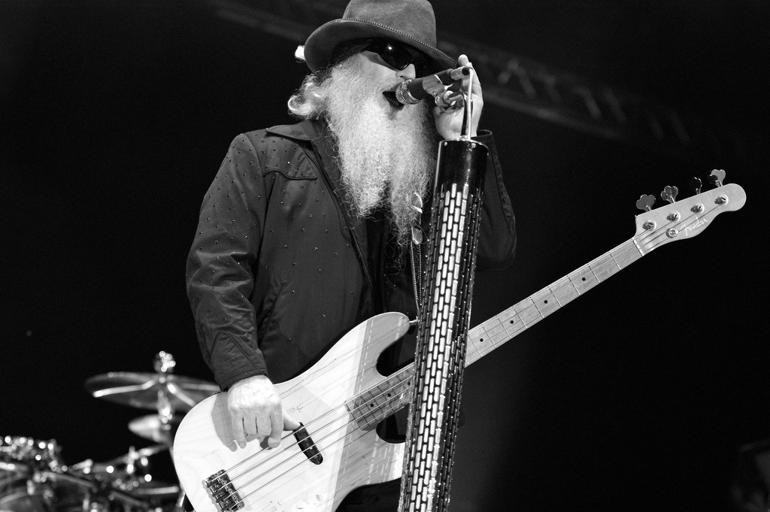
[171, 167, 748, 512]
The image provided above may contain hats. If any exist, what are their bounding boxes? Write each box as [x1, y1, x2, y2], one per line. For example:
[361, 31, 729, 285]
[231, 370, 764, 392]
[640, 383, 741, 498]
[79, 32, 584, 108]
[304, 2, 458, 69]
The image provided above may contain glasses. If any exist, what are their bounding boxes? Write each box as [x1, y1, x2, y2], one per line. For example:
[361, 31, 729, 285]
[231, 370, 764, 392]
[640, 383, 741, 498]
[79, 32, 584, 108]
[327, 34, 441, 77]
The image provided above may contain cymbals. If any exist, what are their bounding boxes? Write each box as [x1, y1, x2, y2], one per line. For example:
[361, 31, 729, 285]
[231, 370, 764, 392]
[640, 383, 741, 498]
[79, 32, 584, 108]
[86, 371, 221, 410]
[129, 414, 184, 445]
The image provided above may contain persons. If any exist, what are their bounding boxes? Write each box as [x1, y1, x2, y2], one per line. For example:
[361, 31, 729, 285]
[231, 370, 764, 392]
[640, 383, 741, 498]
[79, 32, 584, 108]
[183, 0, 518, 512]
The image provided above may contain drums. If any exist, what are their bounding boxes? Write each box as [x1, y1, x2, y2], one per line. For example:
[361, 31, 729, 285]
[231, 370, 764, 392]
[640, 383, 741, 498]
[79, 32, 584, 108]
[0, 460, 155, 511]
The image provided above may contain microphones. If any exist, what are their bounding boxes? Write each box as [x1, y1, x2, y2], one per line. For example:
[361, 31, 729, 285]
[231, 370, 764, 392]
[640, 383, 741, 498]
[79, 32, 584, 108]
[394, 63, 471, 107]
